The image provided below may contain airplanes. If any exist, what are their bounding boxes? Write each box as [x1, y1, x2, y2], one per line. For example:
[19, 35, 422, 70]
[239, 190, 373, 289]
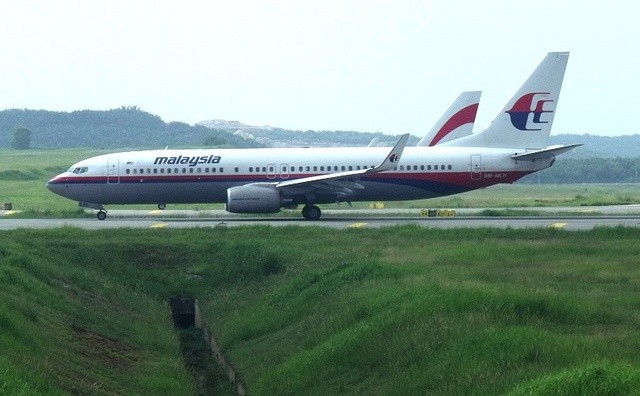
[44, 50, 584, 218]
